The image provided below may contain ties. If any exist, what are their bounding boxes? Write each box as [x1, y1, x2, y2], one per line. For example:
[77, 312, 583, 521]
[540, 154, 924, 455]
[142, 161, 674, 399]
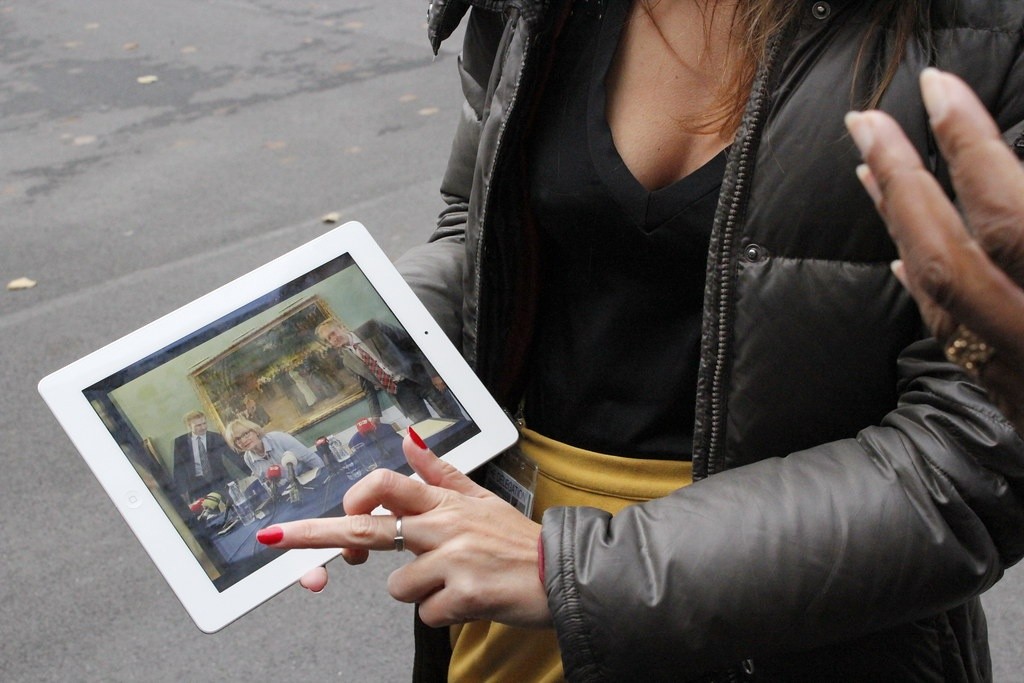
[196, 436, 214, 483]
[353, 344, 397, 394]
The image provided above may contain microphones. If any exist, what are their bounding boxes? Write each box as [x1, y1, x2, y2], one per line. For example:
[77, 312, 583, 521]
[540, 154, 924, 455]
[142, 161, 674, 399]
[355, 417, 381, 448]
[282, 451, 298, 487]
[315, 436, 332, 467]
[198, 492, 221, 523]
[267, 464, 282, 497]
[224, 487, 235, 520]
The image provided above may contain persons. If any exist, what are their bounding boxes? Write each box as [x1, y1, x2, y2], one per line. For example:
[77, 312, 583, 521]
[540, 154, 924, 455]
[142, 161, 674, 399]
[231, 342, 346, 427]
[226, 418, 325, 484]
[172, 411, 252, 504]
[314, 317, 466, 430]
[255, 1, 1023, 682]
[845, 68, 1022, 398]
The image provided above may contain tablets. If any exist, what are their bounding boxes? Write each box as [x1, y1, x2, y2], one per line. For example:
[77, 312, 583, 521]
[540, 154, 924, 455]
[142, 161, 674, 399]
[37, 221, 518, 634]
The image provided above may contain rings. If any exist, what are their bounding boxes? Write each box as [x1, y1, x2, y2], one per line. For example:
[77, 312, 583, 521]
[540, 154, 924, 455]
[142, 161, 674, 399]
[395, 515, 403, 552]
[942, 324, 994, 375]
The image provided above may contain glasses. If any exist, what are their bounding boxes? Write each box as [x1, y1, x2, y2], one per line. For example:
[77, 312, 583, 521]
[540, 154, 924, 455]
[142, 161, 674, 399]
[234, 429, 251, 444]
